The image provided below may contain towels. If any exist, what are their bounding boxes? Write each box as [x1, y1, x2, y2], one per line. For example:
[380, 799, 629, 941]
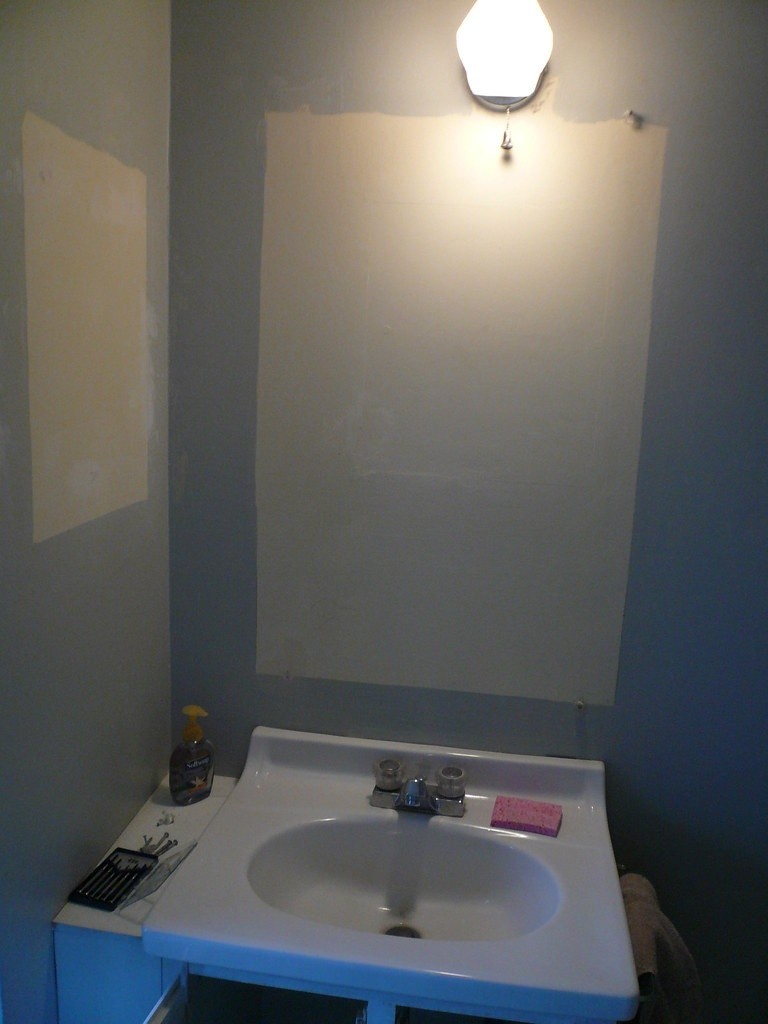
[619, 873, 706, 1024]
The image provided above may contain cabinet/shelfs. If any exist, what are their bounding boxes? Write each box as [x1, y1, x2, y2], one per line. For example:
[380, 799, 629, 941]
[139, 960, 588, 1024]
[255, 109, 664, 709]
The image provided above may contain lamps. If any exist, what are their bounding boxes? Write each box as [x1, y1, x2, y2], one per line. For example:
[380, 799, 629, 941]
[457, 0, 555, 151]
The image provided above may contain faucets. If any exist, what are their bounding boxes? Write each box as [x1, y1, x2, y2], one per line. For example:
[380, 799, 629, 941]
[395, 775, 438, 814]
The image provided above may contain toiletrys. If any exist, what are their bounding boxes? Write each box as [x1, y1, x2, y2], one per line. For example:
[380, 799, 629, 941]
[169, 706, 215, 806]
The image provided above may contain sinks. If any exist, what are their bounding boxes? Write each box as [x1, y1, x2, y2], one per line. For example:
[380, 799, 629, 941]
[247, 813, 560, 937]
